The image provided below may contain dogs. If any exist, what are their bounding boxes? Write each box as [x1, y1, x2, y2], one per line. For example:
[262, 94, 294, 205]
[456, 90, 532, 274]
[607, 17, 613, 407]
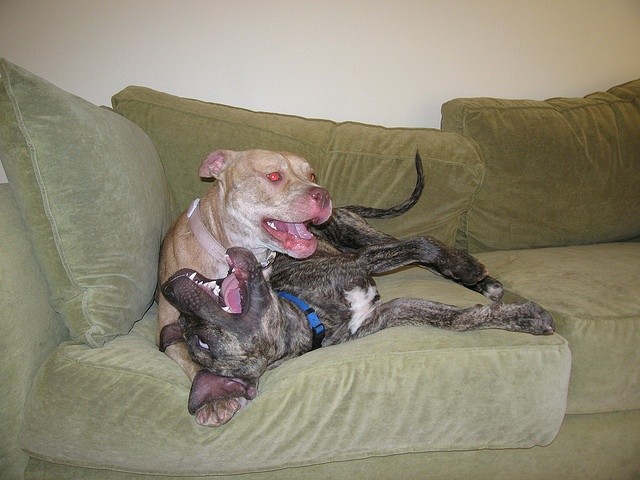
[158, 151, 555, 416]
[152, 148, 333, 428]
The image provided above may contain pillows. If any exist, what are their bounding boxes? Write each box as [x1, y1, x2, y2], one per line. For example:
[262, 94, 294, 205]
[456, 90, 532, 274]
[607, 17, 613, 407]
[110, 86, 484, 256]
[441, 77, 639, 252]
[0, 58, 168, 351]
[2, 85, 640, 478]
